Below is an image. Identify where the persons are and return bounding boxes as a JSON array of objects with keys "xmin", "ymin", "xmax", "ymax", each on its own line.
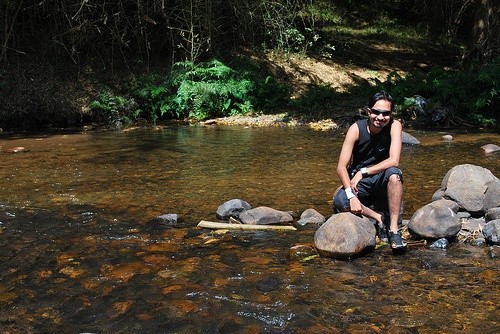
[{"xmin": 332, "ymin": 90, "xmax": 408, "ymax": 250}]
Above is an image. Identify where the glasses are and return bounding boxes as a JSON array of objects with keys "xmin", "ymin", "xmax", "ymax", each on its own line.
[{"xmin": 371, "ymin": 109, "xmax": 391, "ymax": 115}]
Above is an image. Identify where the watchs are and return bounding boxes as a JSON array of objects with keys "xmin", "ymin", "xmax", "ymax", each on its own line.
[{"xmin": 361, "ymin": 167, "xmax": 368, "ymax": 178}]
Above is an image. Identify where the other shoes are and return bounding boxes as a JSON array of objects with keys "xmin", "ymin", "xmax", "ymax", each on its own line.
[
  {"xmin": 378, "ymin": 213, "xmax": 389, "ymax": 242},
  {"xmin": 390, "ymin": 231, "xmax": 407, "ymax": 248}
]
